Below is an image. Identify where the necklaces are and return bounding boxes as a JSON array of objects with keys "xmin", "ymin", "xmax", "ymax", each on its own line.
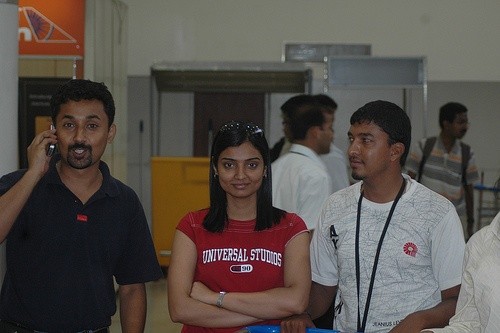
[{"xmin": 355, "ymin": 179, "xmax": 406, "ymax": 333}]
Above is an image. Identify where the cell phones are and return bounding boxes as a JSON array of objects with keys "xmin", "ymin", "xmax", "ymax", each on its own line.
[{"xmin": 46, "ymin": 122, "xmax": 55, "ymax": 156}]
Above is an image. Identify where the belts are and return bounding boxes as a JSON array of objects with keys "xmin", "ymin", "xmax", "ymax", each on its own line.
[{"xmin": 2, "ymin": 320, "xmax": 107, "ymax": 333}]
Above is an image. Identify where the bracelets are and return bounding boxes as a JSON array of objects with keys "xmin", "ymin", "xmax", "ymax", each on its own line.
[
  {"xmin": 217, "ymin": 292, "xmax": 227, "ymax": 308},
  {"xmin": 466, "ymin": 219, "xmax": 475, "ymax": 223}
]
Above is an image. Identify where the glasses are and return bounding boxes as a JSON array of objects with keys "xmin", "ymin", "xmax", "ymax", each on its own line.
[{"xmin": 218, "ymin": 121, "xmax": 263, "ymax": 136}]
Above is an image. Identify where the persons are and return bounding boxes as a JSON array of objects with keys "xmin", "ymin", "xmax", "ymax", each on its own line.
[
  {"xmin": 167, "ymin": 121, "xmax": 311, "ymax": 333},
  {"xmin": 420, "ymin": 211, "xmax": 500, "ymax": 333},
  {"xmin": 272, "ymin": 94, "xmax": 350, "ymax": 241},
  {"xmin": 404, "ymin": 102, "xmax": 479, "ymax": 243},
  {"xmin": 0, "ymin": 79, "xmax": 163, "ymax": 333},
  {"xmin": 281, "ymin": 99, "xmax": 465, "ymax": 333}
]
